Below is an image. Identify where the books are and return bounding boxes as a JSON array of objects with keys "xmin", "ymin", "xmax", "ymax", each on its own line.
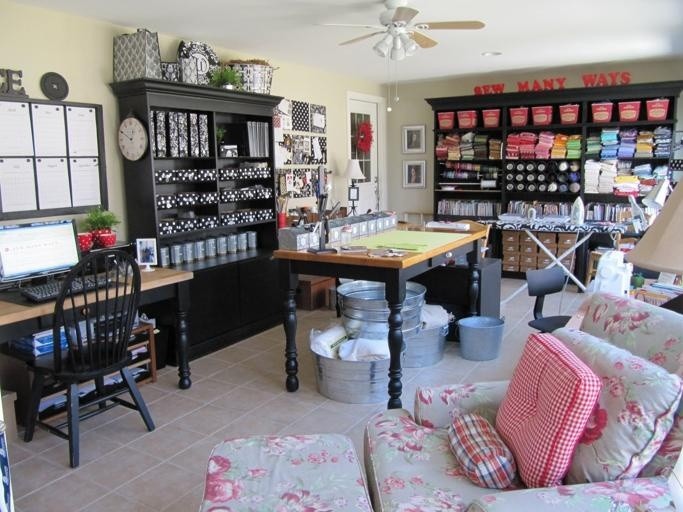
[
  {"xmin": 436, "ymin": 199, "xmax": 658, "ymax": 224},
  {"xmin": 636, "ymin": 282, "xmax": 682, "ymax": 305},
  {"xmin": 237, "ymin": 120, "xmax": 268, "ymax": 158}
]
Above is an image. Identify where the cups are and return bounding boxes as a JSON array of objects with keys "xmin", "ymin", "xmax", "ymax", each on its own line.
[
  {"xmin": 77, "ymin": 232, "xmax": 91, "ymax": 253},
  {"xmin": 277, "ymin": 212, "xmax": 284, "ymax": 228},
  {"xmin": 527, "ymin": 208, "xmax": 536, "ymax": 219}
]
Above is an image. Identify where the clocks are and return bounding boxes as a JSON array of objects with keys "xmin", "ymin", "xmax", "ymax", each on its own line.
[{"xmin": 118, "ymin": 112, "xmax": 148, "ymax": 161}]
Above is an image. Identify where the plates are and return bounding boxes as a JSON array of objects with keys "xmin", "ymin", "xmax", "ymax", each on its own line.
[{"xmin": 497, "ymin": 213, "xmax": 519, "ymax": 222}]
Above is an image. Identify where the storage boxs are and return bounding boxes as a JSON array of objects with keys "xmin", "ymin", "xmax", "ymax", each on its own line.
[{"xmin": 295, "ymin": 273, "xmax": 336, "ymax": 310}]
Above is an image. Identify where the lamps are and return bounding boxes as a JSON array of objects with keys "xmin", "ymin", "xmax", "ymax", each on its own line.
[
  {"xmin": 372, "ymin": 32, "xmax": 419, "ymax": 113},
  {"xmin": 343, "ymin": 160, "xmax": 366, "ymax": 217}
]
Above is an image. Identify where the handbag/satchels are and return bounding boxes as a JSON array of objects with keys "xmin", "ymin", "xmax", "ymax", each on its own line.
[{"xmin": 113, "ymin": 28, "xmax": 162, "ymax": 82}]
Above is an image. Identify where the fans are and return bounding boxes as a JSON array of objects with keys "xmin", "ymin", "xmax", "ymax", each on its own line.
[{"xmin": 324, "ymin": 0, "xmax": 483, "ymax": 48}]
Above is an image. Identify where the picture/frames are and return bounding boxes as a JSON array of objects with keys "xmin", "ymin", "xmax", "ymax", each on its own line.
[
  {"xmin": 402, "ymin": 125, "xmax": 425, "ymax": 154},
  {"xmin": 136, "ymin": 238, "xmax": 157, "ymax": 272},
  {"xmin": 402, "ymin": 160, "xmax": 425, "ymax": 189}
]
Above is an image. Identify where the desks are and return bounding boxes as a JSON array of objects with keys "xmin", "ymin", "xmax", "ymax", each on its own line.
[
  {"xmin": 0, "ymin": 265, "xmax": 194, "ymax": 389},
  {"xmin": 273, "ymin": 223, "xmax": 487, "ymax": 409}
]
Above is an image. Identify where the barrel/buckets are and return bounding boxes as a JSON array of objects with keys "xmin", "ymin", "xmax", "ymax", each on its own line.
[{"xmin": 455, "ymin": 316, "xmax": 507, "ymax": 362}]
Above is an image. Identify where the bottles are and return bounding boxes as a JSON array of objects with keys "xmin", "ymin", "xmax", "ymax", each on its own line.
[
  {"xmin": 572, "ymin": 197, "xmax": 583, "ymax": 225},
  {"xmin": 628, "ymin": 194, "xmax": 647, "ymax": 233},
  {"xmin": 339, "ymin": 227, "xmax": 351, "ymax": 250}
]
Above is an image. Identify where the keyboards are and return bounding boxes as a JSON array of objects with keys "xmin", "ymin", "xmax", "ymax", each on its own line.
[{"xmin": 20, "ymin": 276, "xmax": 111, "ymax": 302}]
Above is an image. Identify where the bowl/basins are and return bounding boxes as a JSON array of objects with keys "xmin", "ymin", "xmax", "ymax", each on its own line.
[
  {"xmin": 292, "ymin": 220, "xmax": 303, "ymax": 227},
  {"xmin": 97, "ymin": 233, "xmax": 116, "ymax": 249}
]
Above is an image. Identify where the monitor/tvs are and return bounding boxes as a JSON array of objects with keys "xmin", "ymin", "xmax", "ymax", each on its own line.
[{"xmin": 0, "ymin": 218, "xmax": 82, "ymax": 292}]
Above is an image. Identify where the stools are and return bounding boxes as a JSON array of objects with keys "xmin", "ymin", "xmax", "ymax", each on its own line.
[{"xmin": 198, "ymin": 433, "xmax": 374, "ymax": 512}]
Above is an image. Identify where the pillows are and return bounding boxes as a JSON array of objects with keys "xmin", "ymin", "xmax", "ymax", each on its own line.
[
  {"xmin": 493, "ymin": 332, "xmax": 602, "ymax": 490},
  {"xmin": 446, "ymin": 412, "xmax": 516, "ymax": 489},
  {"xmin": 551, "ymin": 327, "xmax": 683, "ymax": 485}
]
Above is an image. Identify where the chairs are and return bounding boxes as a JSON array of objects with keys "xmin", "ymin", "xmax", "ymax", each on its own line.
[
  {"xmin": 24, "ymin": 249, "xmax": 155, "ymax": 468},
  {"xmin": 364, "ymin": 292, "xmax": 683, "ymax": 512},
  {"xmin": 524, "ymin": 267, "xmax": 573, "ymax": 331}
]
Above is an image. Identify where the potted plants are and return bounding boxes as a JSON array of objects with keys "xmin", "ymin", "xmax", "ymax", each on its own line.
[
  {"xmin": 80, "ymin": 205, "xmax": 122, "ymax": 245},
  {"xmin": 207, "ymin": 66, "xmax": 244, "ymax": 95}
]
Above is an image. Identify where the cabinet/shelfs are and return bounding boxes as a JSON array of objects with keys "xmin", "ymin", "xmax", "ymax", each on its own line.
[
  {"xmin": 109, "ymin": 77, "xmax": 284, "ymax": 367},
  {"xmin": 424, "ymin": 81, "xmax": 683, "ymax": 303},
  {"xmin": 0, "ymin": 323, "xmax": 157, "ymax": 427},
  {"xmin": 408, "ymin": 257, "xmax": 501, "ymax": 342}
]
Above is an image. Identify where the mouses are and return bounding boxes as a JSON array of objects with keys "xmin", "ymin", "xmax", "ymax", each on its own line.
[{"xmin": 101, "ymin": 275, "xmax": 111, "ymax": 283}]
